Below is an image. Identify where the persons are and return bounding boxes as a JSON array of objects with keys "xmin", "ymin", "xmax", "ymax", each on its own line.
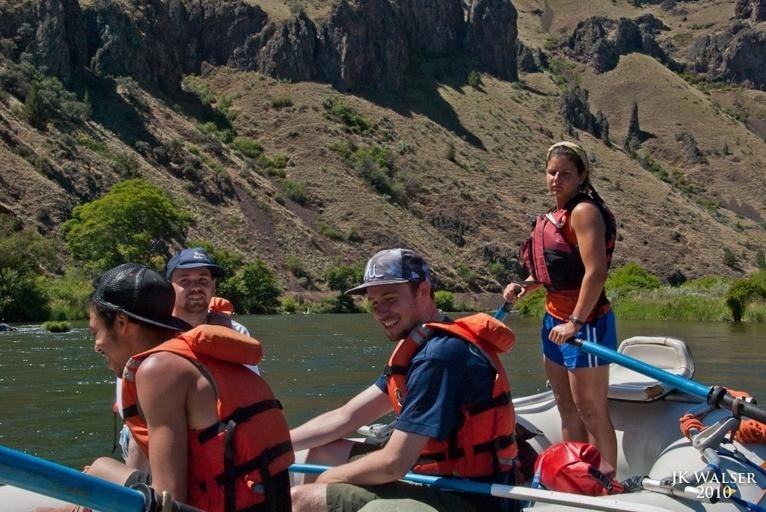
[
  {"xmin": 112, "ymin": 247, "xmax": 261, "ymax": 460},
  {"xmin": 0, "ymin": 259, "xmax": 296, "ymax": 512},
  {"xmin": 290, "ymin": 249, "xmax": 510, "ymax": 512},
  {"xmin": 505, "ymin": 141, "xmax": 617, "ymax": 479}
]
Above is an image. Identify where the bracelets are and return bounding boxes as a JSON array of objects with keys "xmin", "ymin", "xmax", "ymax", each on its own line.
[{"xmin": 568, "ymin": 316, "xmax": 585, "ymax": 326}]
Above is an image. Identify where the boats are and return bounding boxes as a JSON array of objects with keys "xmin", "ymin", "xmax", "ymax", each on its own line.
[{"xmin": 0, "ymin": 336, "xmax": 766, "ymax": 511}]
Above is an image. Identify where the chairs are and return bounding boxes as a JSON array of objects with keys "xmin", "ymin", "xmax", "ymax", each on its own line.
[{"xmin": 608, "ymin": 335, "xmax": 695, "ymax": 402}]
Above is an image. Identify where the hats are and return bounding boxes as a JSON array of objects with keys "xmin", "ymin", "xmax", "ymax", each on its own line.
[
  {"xmin": 167, "ymin": 248, "xmax": 224, "ymax": 280},
  {"xmin": 96, "ymin": 263, "xmax": 193, "ymax": 330},
  {"xmin": 344, "ymin": 248, "xmax": 430, "ymax": 295}
]
